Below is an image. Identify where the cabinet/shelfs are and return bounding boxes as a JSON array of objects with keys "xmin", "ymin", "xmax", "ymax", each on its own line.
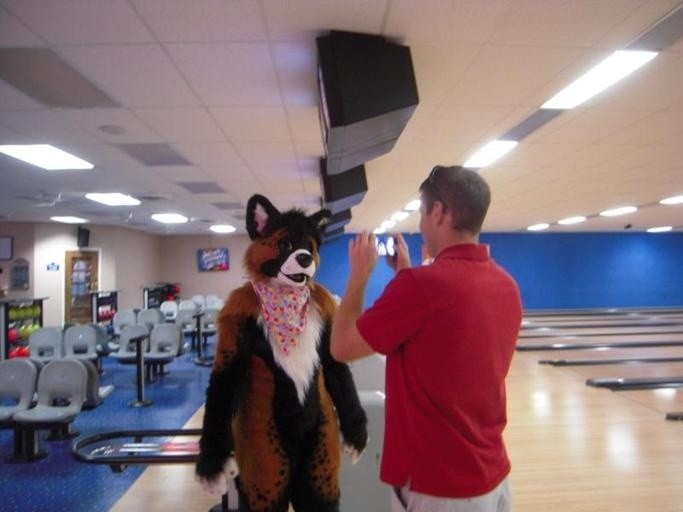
[
  {"xmin": 90, "ymin": 291, "xmax": 118, "ymax": 324},
  {"xmin": 143, "ymin": 284, "xmax": 181, "ymax": 309}
]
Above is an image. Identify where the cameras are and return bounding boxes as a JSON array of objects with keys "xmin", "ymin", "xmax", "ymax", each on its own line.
[{"xmin": 374, "ymin": 234, "xmax": 395, "ymax": 256}]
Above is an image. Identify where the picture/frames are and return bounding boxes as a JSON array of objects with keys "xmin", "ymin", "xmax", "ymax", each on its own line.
[{"xmin": 0, "ymin": 236, "xmax": 13, "ymax": 260}]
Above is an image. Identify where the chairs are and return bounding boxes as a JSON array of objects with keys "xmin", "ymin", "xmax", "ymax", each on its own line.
[{"xmin": 0, "ymin": 295, "xmax": 224, "ymax": 465}]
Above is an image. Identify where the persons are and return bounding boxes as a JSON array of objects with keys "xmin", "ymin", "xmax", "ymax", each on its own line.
[{"xmin": 331, "ymin": 164, "xmax": 525, "ymax": 510}]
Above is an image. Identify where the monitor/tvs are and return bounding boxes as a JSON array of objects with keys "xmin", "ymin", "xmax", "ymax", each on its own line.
[{"xmin": 317, "ymin": 32, "xmax": 421, "ymax": 241}]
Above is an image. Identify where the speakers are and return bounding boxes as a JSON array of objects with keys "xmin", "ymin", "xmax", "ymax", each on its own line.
[{"xmin": 78, "ymin": 228, "xmax": 89, "ymax": 248}]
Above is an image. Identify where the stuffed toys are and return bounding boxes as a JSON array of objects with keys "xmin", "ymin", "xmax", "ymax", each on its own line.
[{"xmin": 193, "ymin": 194, "xmax": 368, "ymax": 512}]
